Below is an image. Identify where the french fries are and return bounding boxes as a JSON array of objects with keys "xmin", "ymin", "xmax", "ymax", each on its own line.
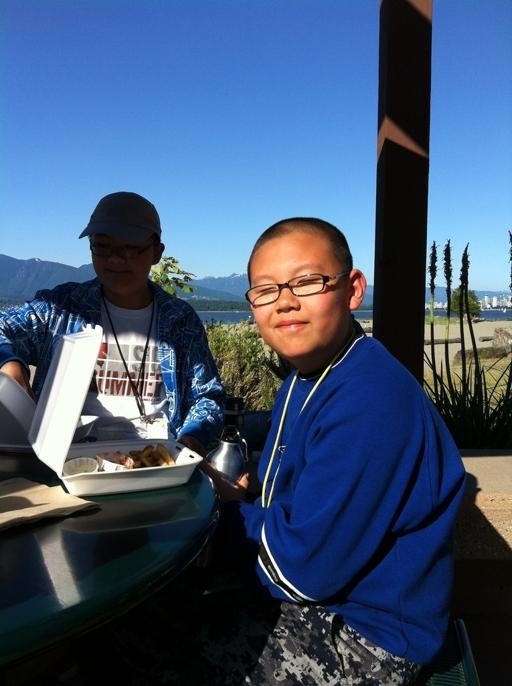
[{"xmin": 129, "ymin": 442, "xmax": 175, "ymax": 468}]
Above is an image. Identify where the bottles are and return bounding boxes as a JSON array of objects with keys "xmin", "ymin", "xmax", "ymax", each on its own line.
[{"xmin": 205, "ymin": 425, "xmax": 248, "ymax": 485}]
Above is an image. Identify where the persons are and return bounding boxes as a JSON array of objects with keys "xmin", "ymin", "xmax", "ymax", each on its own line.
[
  {"xmin": 0, "ymin": 190, "xmax": 230, "ymax": 464},
  {"xmin": 208, "ymin": 216, "xmax": 468, "ymax": 685}
]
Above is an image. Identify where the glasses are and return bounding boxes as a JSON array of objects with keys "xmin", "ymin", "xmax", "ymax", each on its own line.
[
  {"xmin": 88, "ymin": 237, "xmax": 159, "ymax": 258},
  {"xmin": 244, "ymin": 270, "xmax": 353, "ymax": 309}
]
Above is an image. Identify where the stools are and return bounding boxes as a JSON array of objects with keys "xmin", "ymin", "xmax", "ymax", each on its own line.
[{"xmin": 409, "ymin": 619, "xmax": 480, "ymax": 686}]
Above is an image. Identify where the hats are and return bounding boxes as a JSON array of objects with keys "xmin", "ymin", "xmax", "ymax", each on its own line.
[{"xmin": 77, "ymin": 189, "xmax": 164, "ymax": 244}]
